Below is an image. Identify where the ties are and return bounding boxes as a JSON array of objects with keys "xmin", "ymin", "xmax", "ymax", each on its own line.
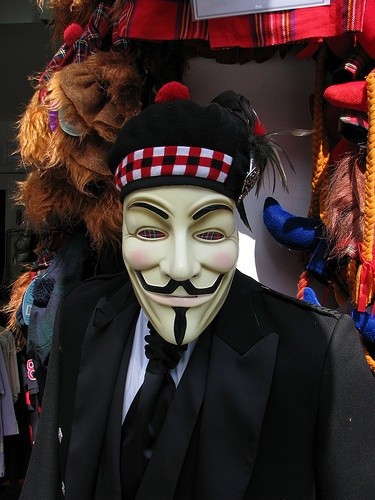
[{"xmin": 119, "ymin": 319, "xmax": 188, "ymax": 499}]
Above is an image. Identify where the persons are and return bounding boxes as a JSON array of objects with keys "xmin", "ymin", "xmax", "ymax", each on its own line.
[{"xmin": 20, "ymin": 82, "xmax": 374, "ymax": 500}]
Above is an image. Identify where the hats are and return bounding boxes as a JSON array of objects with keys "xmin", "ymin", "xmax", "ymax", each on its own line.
[{"xmin": 106, "ymin": 81, "xmax": 298, "ymax": 202}]
